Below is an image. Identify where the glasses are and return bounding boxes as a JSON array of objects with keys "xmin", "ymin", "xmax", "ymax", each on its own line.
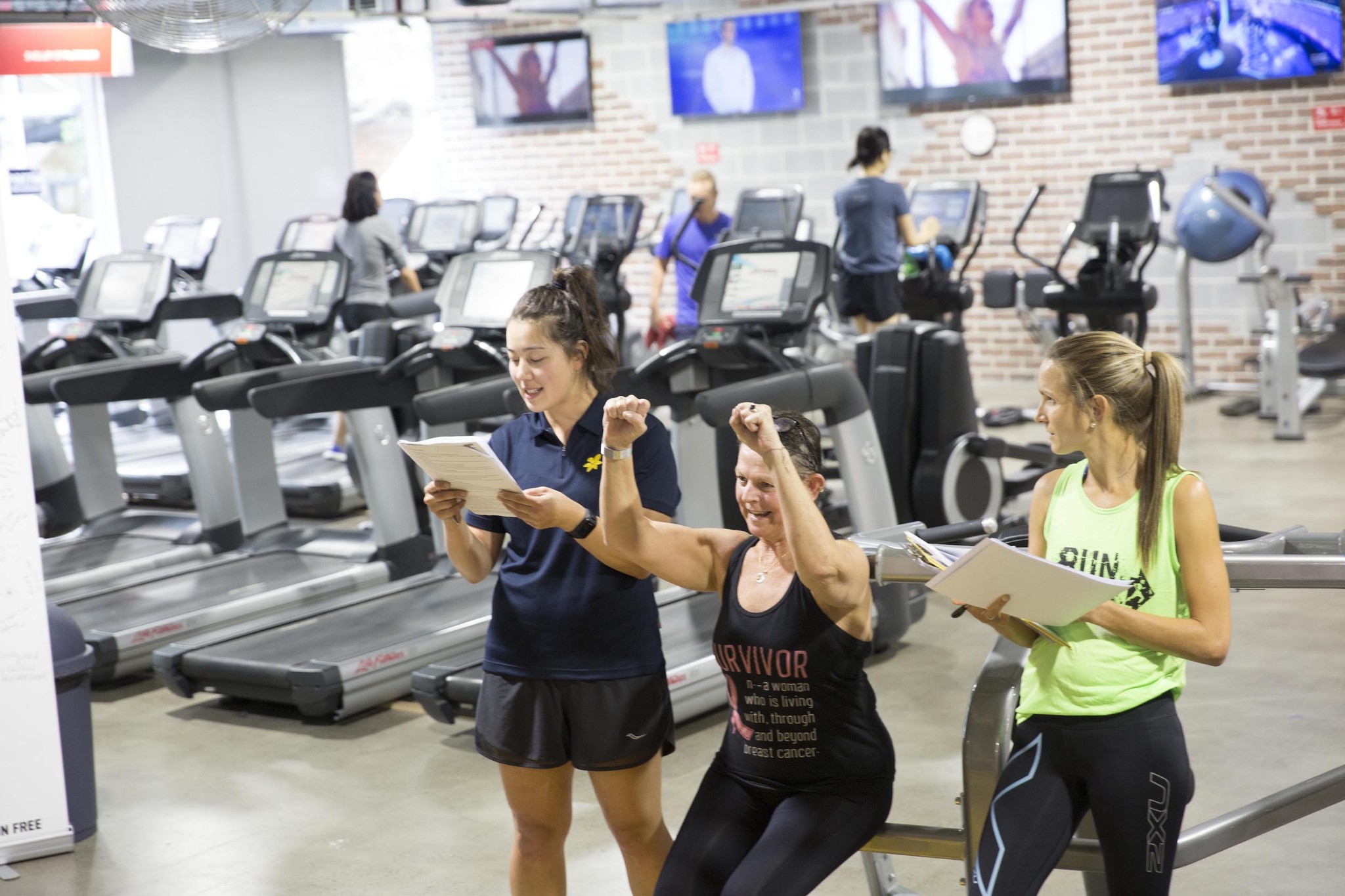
[{"xmin": 774, "ymin": 416, "xmax": 820, "ymax": 470}]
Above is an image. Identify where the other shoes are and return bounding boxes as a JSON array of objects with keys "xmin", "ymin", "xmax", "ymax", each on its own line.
[{"xmin": 324, "ymin": 443, "xmax": 347, "ymax": 462}]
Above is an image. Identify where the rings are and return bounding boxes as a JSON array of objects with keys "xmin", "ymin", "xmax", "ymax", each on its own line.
[{"xmin": 985, "ymin": 615, "xmax": 995, "ymax": 620}]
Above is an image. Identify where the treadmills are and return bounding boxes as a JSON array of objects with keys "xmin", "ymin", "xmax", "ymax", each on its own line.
[
  {"xmin": 14, "ymin": 237, "xmax": 439, "ymax": 688},
  {"xmin": 75, "ymin": 168, "xmax": 1175, "ymax": 551},
  {"xmin": 150, "ymin": 234, "xmax": 630, "ymax": 725},
  {"xmin": 410, "ymin": 231, "xmax": 928, "ymax": 731}
]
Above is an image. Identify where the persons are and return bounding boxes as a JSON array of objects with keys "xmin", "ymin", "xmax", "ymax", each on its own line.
[
  {"xmin": 953, "ymin": 332, "xmax": 1230, "ymax": 896},
  {"xmin": 324, "ymin": 171, "xmax": 421, "ymax": 461},
  {"xmin": 916, "ymin": 0, "xmax": 1025, "ymax": 83},
  {"xmin": 702, "ymin": 21, "xmax": 754, "ymax": 114},
  {"xmin": 835, "ymin": 128, "xmax": 937, "ymax": 338},
  {"xmin": 422, "ymin": 267, "xmax": 683, "ymax": 896},
  {"xmin": 1200, "ymin": 1, "xmax": 1220, "ymax": 51},
  {"xmin": 599, "ymin": 395, "xmax": 895, "ymax": 896},
  {"xmin": 650, "ymin": 170, "xmax": 731, "ymax": 341},
  {"xmin": 489, "ymin": 41, "xmax": 559, "ymax": 114}
]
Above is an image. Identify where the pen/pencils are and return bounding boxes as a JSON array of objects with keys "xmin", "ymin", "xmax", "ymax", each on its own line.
[{"xmin": 951, "ymin": 604, "xmax": 970, "ymax": 618}]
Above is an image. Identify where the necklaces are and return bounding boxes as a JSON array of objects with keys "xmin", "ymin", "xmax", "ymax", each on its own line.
[{"xmin": 755, "ymin": 547, "xmax": 790, "ymax": 583}]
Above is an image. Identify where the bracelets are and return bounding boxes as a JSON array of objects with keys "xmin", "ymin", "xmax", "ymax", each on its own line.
[{"xmin": 601, "ymin": 445, "xmax": 632, "ymax": 460}]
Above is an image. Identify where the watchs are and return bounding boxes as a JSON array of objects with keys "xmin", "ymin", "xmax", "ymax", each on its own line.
[{"xmin": 565, "ymin": 508, "xmax": 596, "ymax": 539}]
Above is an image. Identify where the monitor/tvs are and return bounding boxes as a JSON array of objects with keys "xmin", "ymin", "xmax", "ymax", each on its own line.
[
  {"xmin": 719, "ymin": 250, "xmax": 806, "ymax": 319},
  {"xmin": 470, "ymin": 31, "xmax": 596, "ymax": 126},
  {"xmin": 876, "ymin": 2, "xmax": 1070, "ymax": 107},
  {"xmin": 80, "ymin": 189, "xmax": 548, "ymax": 330},
  {"xmin": 734, "ymin": 188, "xmax": 805, "ymax": 237},
  {"xmin": 571, "ymin": 195, "xmax": 642, "ymax": 253},
  {"xmin": 902, "ymin": 181, "xmax": 980, "ymax": 243},
  {"xmin": 1080, "ymin": 171, "xmax": 1160, "ymax": 238},
  {"xmin": 664, "ymin": 11, "xmax": 806, "ymax": 115}
]
